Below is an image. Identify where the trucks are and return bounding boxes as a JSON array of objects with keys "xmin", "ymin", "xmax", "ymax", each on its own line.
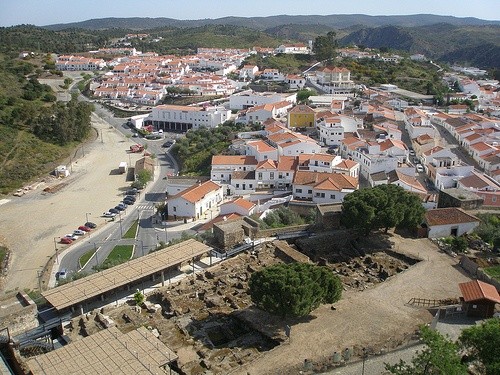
[{"xmin": 119, "ymin": 161, "xmax": 128, "ymax": 173}]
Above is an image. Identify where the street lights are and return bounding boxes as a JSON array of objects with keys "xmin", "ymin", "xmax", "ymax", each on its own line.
[
  {"xmin": 54, "ymin": 237, "xmax": 61, "ymax": 250},
  {"xmin": 86, "ymin": 213, "xmax": 91, "ymax": 222}
]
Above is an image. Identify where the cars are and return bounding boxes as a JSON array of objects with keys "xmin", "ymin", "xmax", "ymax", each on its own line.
[
  {"xmin": 61, "ymin": 238, "xmax": 73, "ymax": 244},
  {"xmin": 64, "ymin": 234, "xmax": 78, "ymax": 240},
  {"xmin": 124, "ymin": 118, "xmax": 167, "ymax": 141},
  {"xmin": 101, "ymin": 94, "xmax": 154, "ymax": 113},
  {"xmin": 72, "ymin": 230, "xmax": 85, "ymax": 236},
  {"xmin": 59, "ymin": 268, "xmax": 68, "ymax": 280},
  {"xmin": 126, "ymin": 185, "xmax": 141, "ymax": 196},
  {"xmin": 129, "ymin": 143, "xmax": 144, "ymax": 153},
  {"xmin": 293, "ymin": 126, "xmax": 341, "ymax": 154},
  {"xmin": 127, "ymin": 195, "xmax": 136, "ymax": 200},
  {"xmin": 85, "ymin": 222, "xmax": 97, "ymax": 228},
  {"xmin": 123, "ymin": 197, "xmax": 135, "ymax": 202},
  {"xmin": 109, "ymin": 209, "xmax": 120, "ymax": 214},
  {"xmin": 123, "ymin": 200, "xmax": 134, "ymax": 205},
  {"xmin": 78, "ymin": 225, "xmax": 91, "ymax": 232},
  {"xmin": 410, "ymin": 149, "xmax": 434, "ymax": 192},
  {"xmin": 118, "ymin": 203, "xmax": 128, "ymax": 208},
  {"xmin": 115, "ymin": 206, "xmax": 125, "ymax": 211},
  {"xmin": 103, "ymin": 212, "xmax": 116, "ymax": 217}
]
